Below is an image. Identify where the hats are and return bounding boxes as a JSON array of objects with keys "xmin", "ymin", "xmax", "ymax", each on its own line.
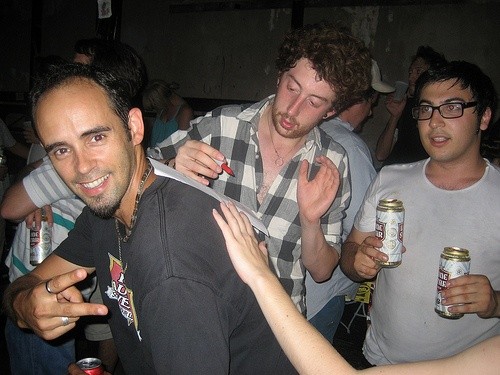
[{"xmin": 368, "ymin": 59, "xmax": 395, "ymax": 93}]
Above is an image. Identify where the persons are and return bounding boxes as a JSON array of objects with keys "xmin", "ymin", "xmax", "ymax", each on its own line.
[{"xmin": 0, "ymin": 25, "xmax": 499, "ymax": 375}]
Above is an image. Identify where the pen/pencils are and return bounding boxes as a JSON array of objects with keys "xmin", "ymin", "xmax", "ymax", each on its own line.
[{"xmin": 210, "ymin": 157, "xmax": 235, "ymax": 177}]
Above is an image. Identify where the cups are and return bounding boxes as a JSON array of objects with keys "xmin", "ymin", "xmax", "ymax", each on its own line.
[{"xmin": 394, "ymin": 80, "xmax": 408, "ymax": 102}]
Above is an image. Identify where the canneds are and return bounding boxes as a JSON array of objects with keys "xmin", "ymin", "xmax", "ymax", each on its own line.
[
  {"xmin": 75, "ymin": 357, "xmax": 104, "ymax": 375},
  {"xmin": 29, "ymin": 214, "xmax": 53, "ymax": 266},
  {"xmin": 373, "ymin": 199, "xmax": 404, "ymax": 268},
  {"xmin": 435, "ymin": 246, "xmax": 471, "ymax": 319}
]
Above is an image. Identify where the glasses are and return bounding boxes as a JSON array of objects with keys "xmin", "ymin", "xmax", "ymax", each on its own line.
[{"xmin": 411, "ymin": 101, "xmax": 478, "ymax": 120}]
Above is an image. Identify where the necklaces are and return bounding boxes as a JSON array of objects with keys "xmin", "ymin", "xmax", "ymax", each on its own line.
[
  {"xmin": 267, "ymin": 107, "xmax": 303, "ymax": 166},
  {"xmin": 116, "ymin": 160, "xmax": 150, "ymax": 243}
]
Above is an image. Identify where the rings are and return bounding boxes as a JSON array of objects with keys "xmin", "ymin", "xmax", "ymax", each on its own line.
[
  {"xmin": 62, "ymin": 316, "xmax": 69, "ymax": 326},
  {"xmin": 45, "ymin": 279, "xmax": 55, "ymax": 293}
]
